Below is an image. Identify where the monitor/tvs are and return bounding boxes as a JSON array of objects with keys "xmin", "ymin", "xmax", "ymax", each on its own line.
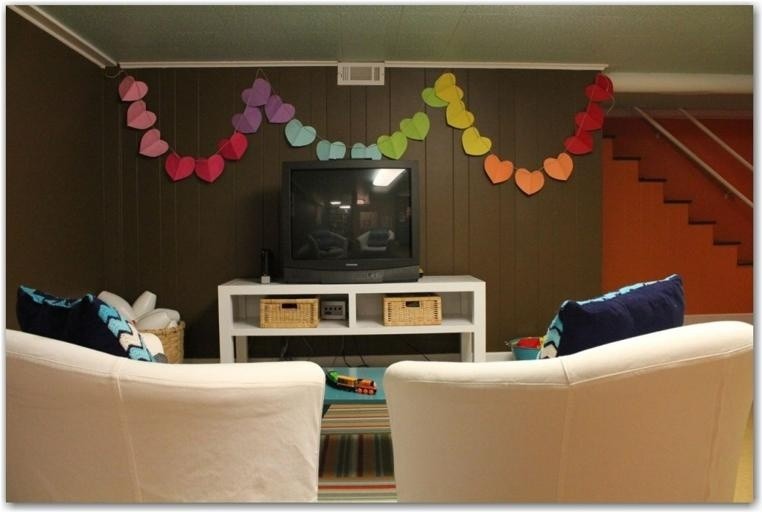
[{"xmin": 280, "ymin": 159, "xmax": 420, "ymax": 284}]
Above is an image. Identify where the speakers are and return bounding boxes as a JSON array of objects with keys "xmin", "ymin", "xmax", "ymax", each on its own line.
[{"xmin": 255, "ymin": 247, "xmax": 271, "ymax": 277}]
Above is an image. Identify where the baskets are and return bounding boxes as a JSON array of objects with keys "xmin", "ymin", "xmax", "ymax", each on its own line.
[
  {"xmin": 258, "ymin": 297, "xmax": 319, "ymax": 328},
  {"xmin": 382, "ymin": 294, "xmax": 441, "ymax": 325},
  {"xmin": 141, "ymin": 318, "xmax": 186, "ymax": 365}
]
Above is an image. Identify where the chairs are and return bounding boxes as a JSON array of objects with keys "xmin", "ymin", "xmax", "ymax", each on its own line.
[
  {"xmin": 5, "ymin": 322, "xmax": 325, "ymax": 501},
  {"xmin": 383, "ymin": 320, "xmax": 756, "ymax": 505}
]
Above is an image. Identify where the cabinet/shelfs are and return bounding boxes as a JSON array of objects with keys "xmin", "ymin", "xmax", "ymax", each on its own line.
[{"xmin": 216, "ymin": 275, "xmax": 490, "ymax": 368}]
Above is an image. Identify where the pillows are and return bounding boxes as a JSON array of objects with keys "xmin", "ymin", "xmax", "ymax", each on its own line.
[
  {"xmin": 535, "ymin": 273, "xmax": 684, "ymax": 363},
  {"xmin": 10, "ymin": 283, "xmax": 160, "ymax": 366}
]
